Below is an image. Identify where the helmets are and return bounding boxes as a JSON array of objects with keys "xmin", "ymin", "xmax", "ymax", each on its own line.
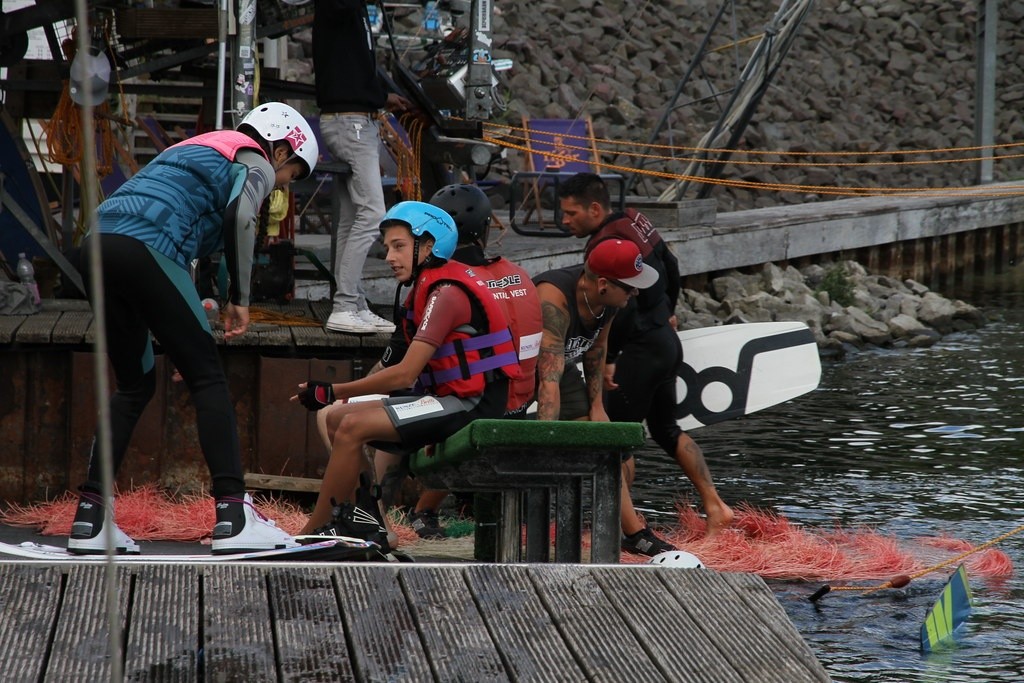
[
  {"xmin": 429, "ymin": 181, "xmax": 491, "ymax": 247},
  {"xmin": 238, "ymin": 102, "xmax": 319, "ymax": 184},
  {"xmin": 379, "ymin": 200, "xmax": 458, "ymax": 264}
]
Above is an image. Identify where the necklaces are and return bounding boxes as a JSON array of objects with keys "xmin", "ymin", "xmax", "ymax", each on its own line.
[{"xmin": 581, "ymin": 274, "xmax": 606, "ymax": 320}]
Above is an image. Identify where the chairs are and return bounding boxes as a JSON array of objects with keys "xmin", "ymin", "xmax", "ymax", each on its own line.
[{"xmin": 0, "ymin": 102, "xmax": 625, "ymax": 298}]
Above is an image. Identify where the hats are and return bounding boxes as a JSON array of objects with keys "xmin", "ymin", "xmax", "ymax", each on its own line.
[
  {"xmin": 70, "ymin": 45, "xmax": 112, "ymax": 107},
  {"xmin": 589, "ymin": 239, "xmax": 659, "ymax": 290}
]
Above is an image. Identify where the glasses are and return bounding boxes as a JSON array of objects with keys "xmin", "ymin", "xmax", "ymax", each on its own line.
[{"xmin": 609, "ymin": 280, "xmax": 633, "ymax": 295}]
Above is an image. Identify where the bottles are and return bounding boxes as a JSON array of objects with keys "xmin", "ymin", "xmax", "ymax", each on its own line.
[
  {"xmin": 16, "ymin": 253, "xmax": 42, "ymax": 306},
  {"xmin": 199, "ymin": 299, "xmax": 221, "ymax": 323}
]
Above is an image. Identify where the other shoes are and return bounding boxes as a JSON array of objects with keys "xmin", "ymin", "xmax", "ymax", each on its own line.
[
  {"xmin": 623, "ymin": 526, "xmax": 678, "ymax": 560},
  {"xmin": 411, "ymin": 512, "xmax": 447, "ymax": 538}
]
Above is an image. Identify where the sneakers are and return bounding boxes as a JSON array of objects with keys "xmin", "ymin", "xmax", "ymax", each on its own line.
[
  {"xmin": 211, "ymin": 495, "xmax": 303, "ymax": 550},
  {"xmin": 326, "ymin": 310, "xmax": 396, "ymax": 334},
  {"xmin": 66, "ymin": 490, "xmax": 142, "ymax": 555}
]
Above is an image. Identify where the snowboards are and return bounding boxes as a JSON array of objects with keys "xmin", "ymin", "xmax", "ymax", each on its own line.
[{"xmin": 0, "ymin": 528, "xmax": 383, "ymax": 563}]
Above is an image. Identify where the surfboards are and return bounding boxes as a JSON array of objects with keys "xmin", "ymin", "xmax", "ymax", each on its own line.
[{"xmin": 347, "ymin": 320, "xmax": 821, "ymax": 439}]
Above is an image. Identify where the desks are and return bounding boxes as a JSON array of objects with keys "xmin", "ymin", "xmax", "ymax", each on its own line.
[{"xmin": 509, "ymin": 170, "xmax": 626, "ymax": 237}]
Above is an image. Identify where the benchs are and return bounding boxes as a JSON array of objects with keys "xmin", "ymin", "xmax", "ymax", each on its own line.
[{"xmin": 407, "ymin": 419, "xmax": 646, "ymax": 565}]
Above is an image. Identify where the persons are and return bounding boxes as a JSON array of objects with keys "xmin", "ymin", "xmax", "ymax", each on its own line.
[
  {"xmin": 559, "ymin": 171, "xmax": 733, "ymax": 540},
  {"xmin": 312, "ymin": 0, "xmax": 413, "ymax": 334},
  {"xmin": 290, "ymin": 200, "xmax": 526, "ymax": 549},
  {"xmin": 68, "ymin": 102, "xmax": 319, "ymax": 556},
  {"xmin": 315, "ymin": 184, "xmax": 680, "ymax": 558}
]
